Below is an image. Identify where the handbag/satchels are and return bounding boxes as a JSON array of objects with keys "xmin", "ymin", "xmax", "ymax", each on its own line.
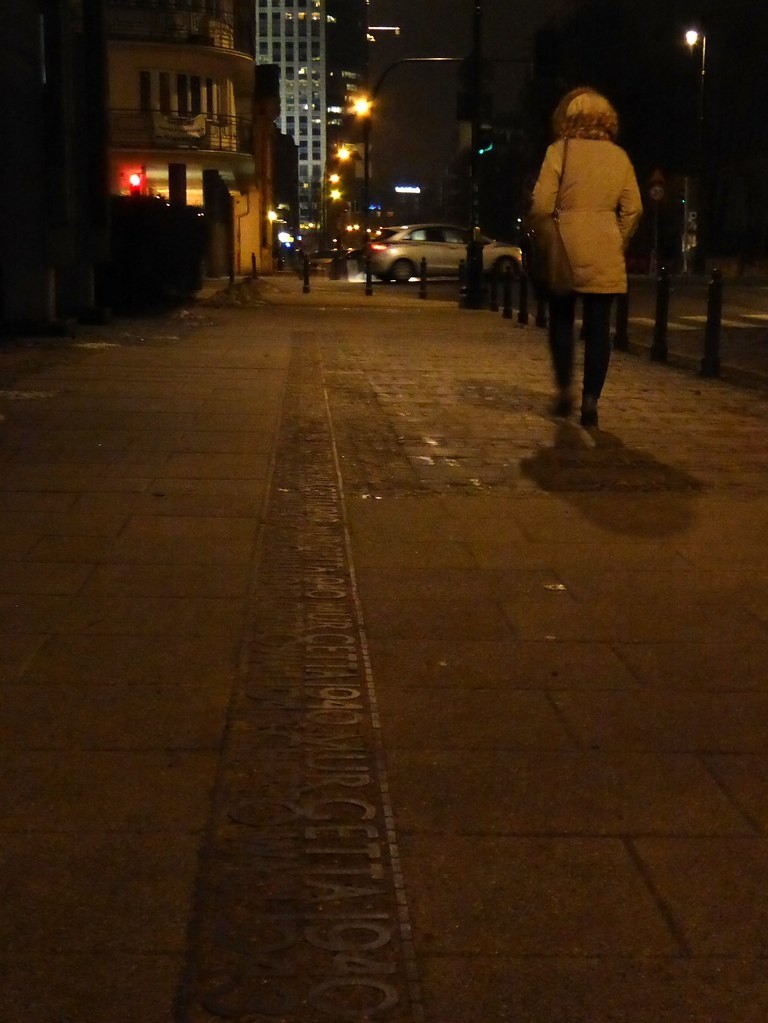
[{"xmin": 517, "ymin": 136, "xmax": 574, "ymax": 297}]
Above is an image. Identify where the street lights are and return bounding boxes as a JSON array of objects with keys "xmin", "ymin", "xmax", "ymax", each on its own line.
[
  {"xmin": 349, "ymin": 93, "xmax": 373, "ymax": 296},
  {"xmin": 336, "ymin": 146, "xmax": 358, "ymax": 258},
  {"xmin": 686, "ymin": 27, "xmax": 708, "ymax": 278}
]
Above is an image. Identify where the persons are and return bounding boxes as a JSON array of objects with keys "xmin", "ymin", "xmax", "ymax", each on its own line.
[{"xmin": 531, "ymin": 87, "xmax": 643, "ymax": 426}]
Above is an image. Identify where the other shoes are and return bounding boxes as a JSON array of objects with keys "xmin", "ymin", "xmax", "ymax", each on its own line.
[
  {"xmin": 552, "ymin": 390, "xmax": 570, "ymax": 416},
  {"xmin": 579, "ymin": 394, "xmax": 599, "ymax": 426}
]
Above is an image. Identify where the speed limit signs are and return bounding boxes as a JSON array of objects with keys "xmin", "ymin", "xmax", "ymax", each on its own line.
[{"xmin": 648, "ymin": 186, "xmax": 665, "ymax": 200}]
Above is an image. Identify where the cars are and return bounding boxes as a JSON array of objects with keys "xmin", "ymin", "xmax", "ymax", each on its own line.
[
  {"xmin": 367, "ymin": 224, "xmax": 522, "ymax": 284},
  {"xmin": 295, "ymin": 247, "xmax": 361, "ymax": 279}
]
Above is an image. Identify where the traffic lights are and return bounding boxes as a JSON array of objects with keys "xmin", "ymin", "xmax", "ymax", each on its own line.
[{"xmin": 478, "ymin": 139, "xmax": 496, "ymax": 162}]
